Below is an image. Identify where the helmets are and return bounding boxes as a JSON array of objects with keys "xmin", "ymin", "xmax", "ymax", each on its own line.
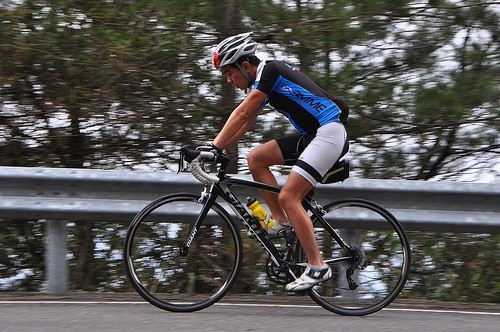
[{"xmin": 214, "ymin": 32, "xmax": 257, "ymax": 69}]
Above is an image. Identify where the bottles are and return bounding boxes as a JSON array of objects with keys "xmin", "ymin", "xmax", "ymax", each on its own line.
[{"xmin": 246, "ymin": 196, "xmax": 274, "ymax": 232}]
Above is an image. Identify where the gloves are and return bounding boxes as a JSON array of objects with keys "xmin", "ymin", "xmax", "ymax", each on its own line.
[{"xmin": 196, "ymin": 143, "xmax": 222, "ymax": 164}]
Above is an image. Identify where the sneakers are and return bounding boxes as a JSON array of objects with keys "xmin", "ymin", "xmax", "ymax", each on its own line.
[
  {"xmin": 247, "ymin": 220, "xmax": 292, "ymax": 238},
  {"xmin": 285, "ymin": 264, "xmax": 332, "ymax": 292}
]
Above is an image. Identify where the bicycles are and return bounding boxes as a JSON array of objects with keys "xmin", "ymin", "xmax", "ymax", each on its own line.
[{"xmin": 123, "ymin": 145, "xmax": 411, "ymax": 316}]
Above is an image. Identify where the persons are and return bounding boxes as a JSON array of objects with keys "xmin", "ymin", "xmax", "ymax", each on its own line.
[{"xmin": 195, "ymin": 32, "xmax": 349, "ymax": 292}]
